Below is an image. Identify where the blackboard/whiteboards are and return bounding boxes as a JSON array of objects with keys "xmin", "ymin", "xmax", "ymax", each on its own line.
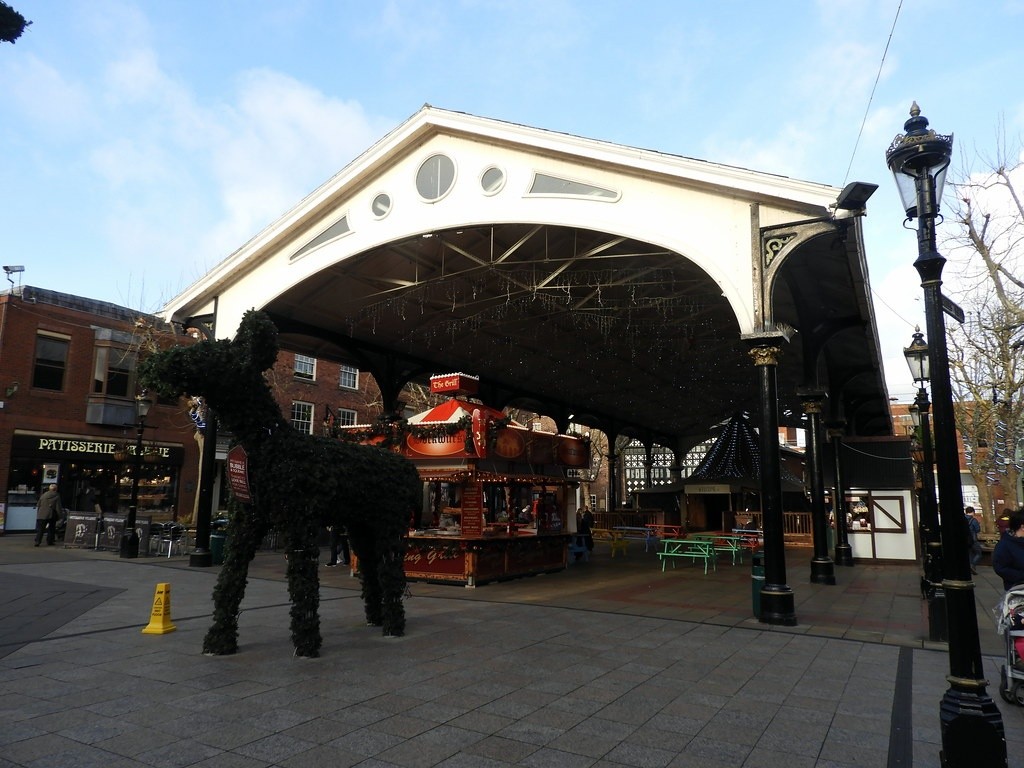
[{"xmin": 536, "ymin": 504, "xmax": 564, "ymax": 536}]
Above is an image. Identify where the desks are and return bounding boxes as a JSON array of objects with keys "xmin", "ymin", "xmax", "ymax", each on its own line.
[{"xmin": 565, "ymin": 523, "xmax": 765, "ymax": 575}]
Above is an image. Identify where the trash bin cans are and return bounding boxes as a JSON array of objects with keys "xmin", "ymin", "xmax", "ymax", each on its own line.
[
  {"xmin": 750, "ymin": 552, "xmax": 766, "ymax": 619},
  {"xmin": 208, "ymin": 520, "xmax": 228, "ymax": 565}
]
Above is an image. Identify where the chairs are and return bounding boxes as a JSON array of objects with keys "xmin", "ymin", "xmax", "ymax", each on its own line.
[{"xmin": 148, "ymin": 521, "xmax": 197, "ymax": 559}]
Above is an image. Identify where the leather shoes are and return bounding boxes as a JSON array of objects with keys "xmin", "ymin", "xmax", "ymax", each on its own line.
[
  {"xmin": 969, "ymin": 565, "xmax": 977, "ymax": 576},
  {"xmin": 34, "ymin": 542, "xmax": 56, "ymax": 546}
]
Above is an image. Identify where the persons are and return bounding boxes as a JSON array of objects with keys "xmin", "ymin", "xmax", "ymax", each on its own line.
[
  {"xmin": 574, "ymin": 508, "xmax": 584, "ymax": 528},
  {"xmin": 325, "ymin": 525, "xmax": 349, "ymax": 567},
  {"xmin": 33, "ymin": 484, "xmax": 63, "ymax": 545},
  {"xmin": 995, "ymin": 508, "xmax": 1015, "ymax": 534},
  {"xmin": 582, "ymin": 505, "xmax": 595, "ymax": 527},
  {"xmin": 962, "ymin": 505, "xmax": 984, "ymax": 574},
  {"xmin": 991, "ymin": 511, "xmax": 1024, "ymax": 593}
]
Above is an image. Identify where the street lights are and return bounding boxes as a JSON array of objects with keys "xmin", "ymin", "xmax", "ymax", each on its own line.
[
  {"xmin": 906, "ymin": 398, "xmax": 935, "ymax": 599},
  {"xmin": 115, "ymin": 388, "xmax": 154, "ymax": 557},
  {"xmin": 903, "ymin": 327, "xmax": 950, "ymax": 638},
  {"xmin": 888, "ymin": 101, "xmax": 1010, "ymax": 768}
]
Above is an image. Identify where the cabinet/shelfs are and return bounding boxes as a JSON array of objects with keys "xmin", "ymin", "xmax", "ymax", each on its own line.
[{"xmin": 118, "ymin": 477, "xmax": 176, "ymax": 516}]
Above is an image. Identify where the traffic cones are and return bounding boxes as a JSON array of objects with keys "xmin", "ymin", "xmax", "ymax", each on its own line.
[{"xmin": 142, "ymin": 582, "xmax": 178, "ymax": 634}]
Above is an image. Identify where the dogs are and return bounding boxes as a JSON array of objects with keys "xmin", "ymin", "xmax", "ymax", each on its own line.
[{"xmin": 137, "ymin": 310, "xmax": 420, "ymax": 658}]
